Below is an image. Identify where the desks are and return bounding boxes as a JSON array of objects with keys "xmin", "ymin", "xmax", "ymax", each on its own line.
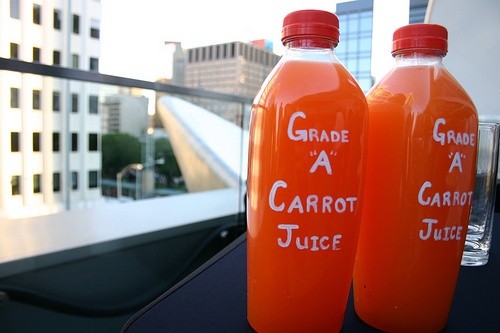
[{"xmin": 119, "ymin": 174, "xmax": 500, "ymax": 333}]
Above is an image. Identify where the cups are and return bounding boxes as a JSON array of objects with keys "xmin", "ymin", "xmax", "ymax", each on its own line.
[{"xmin": 460, "ymin": 123, "xmax": 499, "ymax": 267}]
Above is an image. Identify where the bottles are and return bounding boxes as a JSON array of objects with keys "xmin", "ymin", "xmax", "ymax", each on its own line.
[
  {"xmin": 246, "ymin": 8, "xmax": 370, "ymax": 333},
  {"xmin": 351, "ymin": 23, "xmax": 479, "ymax": 333}
]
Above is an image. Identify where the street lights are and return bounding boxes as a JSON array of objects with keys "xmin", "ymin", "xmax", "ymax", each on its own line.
[{"xmin": 116, "ymin": 163, "xmax": 143, "ymax": 203}]
